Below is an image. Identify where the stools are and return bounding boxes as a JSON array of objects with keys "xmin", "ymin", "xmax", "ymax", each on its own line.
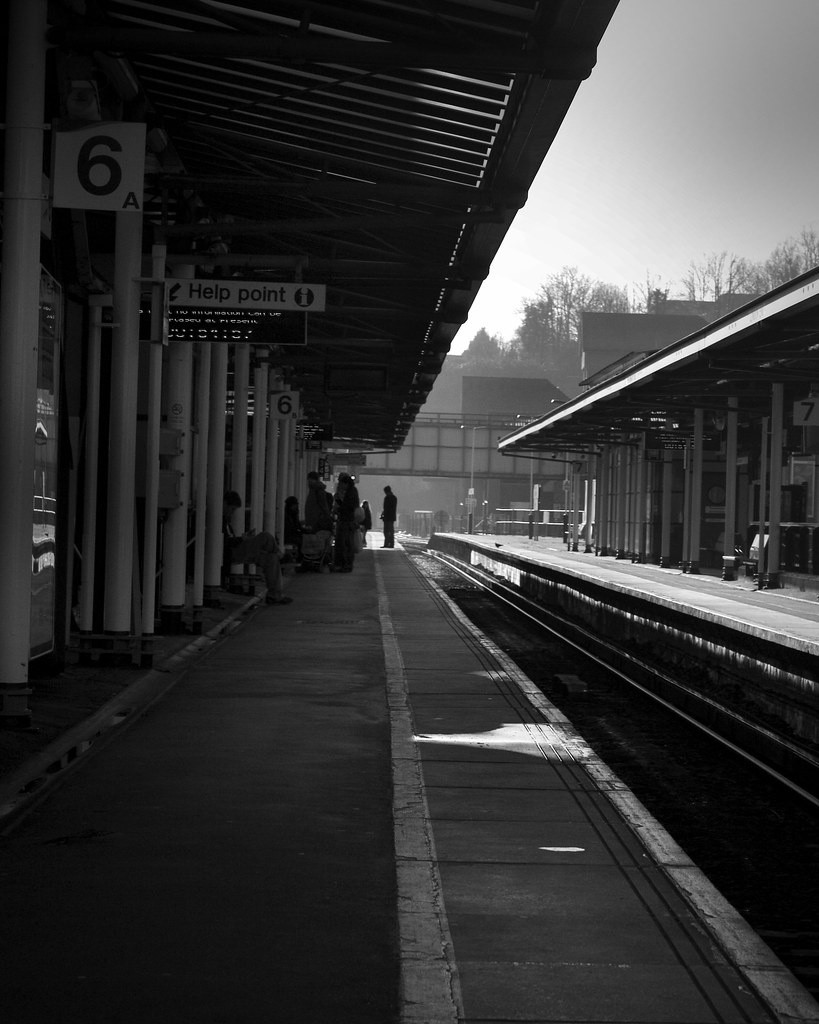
[{"xmin": 228, "ymin": 573, "xmax": 262, "ymax": 596}]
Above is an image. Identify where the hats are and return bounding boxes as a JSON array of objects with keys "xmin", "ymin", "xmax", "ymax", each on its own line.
[{"xmin": 286, "ymin": 496, "xmax": 299, "ymax": 505}]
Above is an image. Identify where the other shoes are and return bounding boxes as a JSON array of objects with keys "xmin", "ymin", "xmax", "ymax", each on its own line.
[
  {"xmin": 266, "ymin": 595, "xmax": 293, "ymax": 605},
  {"xmin": 330, "ymin": 564, "xmax": 351, "ymax": 573}
]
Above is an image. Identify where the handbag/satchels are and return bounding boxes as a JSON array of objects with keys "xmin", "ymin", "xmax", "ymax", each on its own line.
[{"xmin": 354, "ymin": 508, "xmax": 365, "ymax": 523}]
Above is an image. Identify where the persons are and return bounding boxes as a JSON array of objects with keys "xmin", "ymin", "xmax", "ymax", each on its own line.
[
  {"xmin": 362, "ymin": 501, "xmax": 372, "ymax": 546},
  {"xmin": 380, "ymin": 485, "xmax": 397, "ymax": 548},
  {"xmin": 330, "ymin": 471, "xmax": 358, "ymax": 573},
  {"xmin": 284, "ymin": 496, "xmax": 300, "ymax": 550},
  {"xmin": 304, "ymin": 471, "xmax": 333, "ymax": 531},
  {"xmin": 223, "ymin": 491, "xmax": 295, "ymax": 604}
]
